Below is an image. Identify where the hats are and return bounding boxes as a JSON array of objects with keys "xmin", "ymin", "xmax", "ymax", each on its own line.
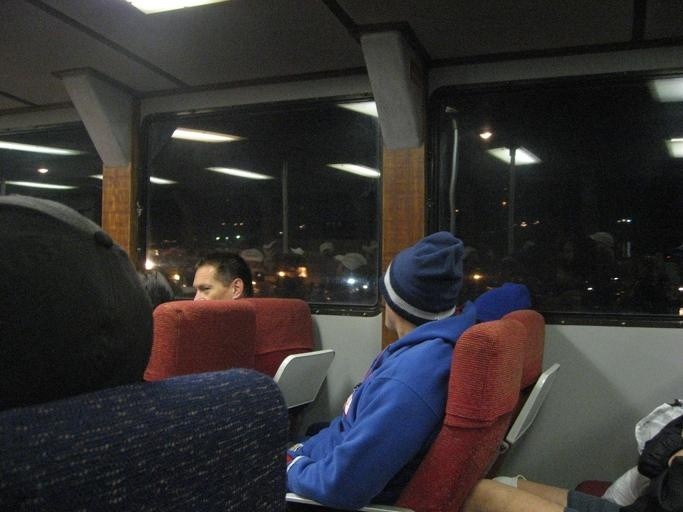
[{"xmin": 380, "ymin": 231, "xmax": 466, "ymax": 324}]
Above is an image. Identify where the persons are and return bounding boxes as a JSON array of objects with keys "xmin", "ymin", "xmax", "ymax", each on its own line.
[
  {"xmin": 0, "ymin": 188, "xmax": 153, "ymax": 410},
  {"xmin": 285, "ymin": 231, "xmax": 532, "ymax": 511},
  {"xmin": 192, "ymin": 251, "xmax": 254, "ymax": 300},
  {"xmin": 138, "ymin": 270, "xmax": 175, "ymax": 307},
  {"xmin": 460, "ymin": 415, "xmax": 683, "ymax": 511}
]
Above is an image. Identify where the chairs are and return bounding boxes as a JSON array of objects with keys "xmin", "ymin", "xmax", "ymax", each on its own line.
[{"xmin": 0, "ymin": 295, "xmax": 551, "ymax": 512}]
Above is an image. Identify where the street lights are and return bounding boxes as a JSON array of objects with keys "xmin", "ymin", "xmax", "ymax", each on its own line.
[{"xmin": 479, "ymin": 130, "xmax": 515, "ymax": 257}]
